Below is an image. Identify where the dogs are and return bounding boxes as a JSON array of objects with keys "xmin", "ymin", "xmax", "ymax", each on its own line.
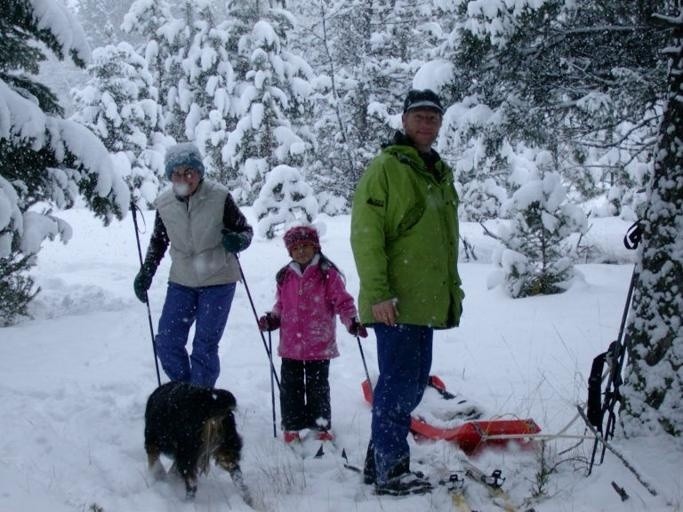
[{"xmin": 142, "ymin": 379, "xmax": 255, "ymax": 512}]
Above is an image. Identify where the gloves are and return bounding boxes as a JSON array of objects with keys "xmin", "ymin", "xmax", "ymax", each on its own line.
[
  {"xmin": 133, "ymin": 263, "xmax": 157, "ymax": 303},
  {"xmin": 260, "ymin": 316, "xmax": 277, "ymax": 331},
  {"xmin": 222, "ymin": 231, "xmax": 245, "ymax": 253},
  {"xmin": 349, "ymin": 322, "xmax": 368, "ymax": 337}
]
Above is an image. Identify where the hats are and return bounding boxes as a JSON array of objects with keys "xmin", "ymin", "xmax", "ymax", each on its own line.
[
  {"xmin": 165, "ymin": 143, "xmax": 205, "ymax": 181},
  {"xmin": 404, "ymin": 89, "xmax": 444, "ymax": 115},
  {"xmin": 283, "ymin": 225, "xmax": 320, "ymax": 257}
]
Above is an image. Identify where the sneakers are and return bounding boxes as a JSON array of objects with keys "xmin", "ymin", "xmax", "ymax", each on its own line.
[
  {"xmin": 283, "ymin": 431, "xmax": 304, "ymax": 448},
  {"xmin": 316, "ymin": 431, "xmax": 336, "ymax": 441},
  {"xmin": 364, "ymin": 471, "xmax": 434, "ymax": 496}
]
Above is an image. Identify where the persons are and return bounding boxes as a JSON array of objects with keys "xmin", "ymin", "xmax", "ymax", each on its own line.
[
  {"xmin": 133, "ymin": 141, "xmax": 254, "ymax": 389},
  {"xmin": 259, "ymin": 226, "xmax": 368, "ymax": 445},
  {"xmin": 350, "ymin": 90, "xmax": 464, "ymax": 496}
]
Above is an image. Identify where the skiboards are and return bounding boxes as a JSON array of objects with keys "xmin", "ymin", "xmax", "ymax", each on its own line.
[
  {"xmin": 288, "ymin": 430, "xmax": 361, "ymax": 475},
  {"xmin": 429, "ymin": 451, "xmax": 523, "ymax": 508}
]
